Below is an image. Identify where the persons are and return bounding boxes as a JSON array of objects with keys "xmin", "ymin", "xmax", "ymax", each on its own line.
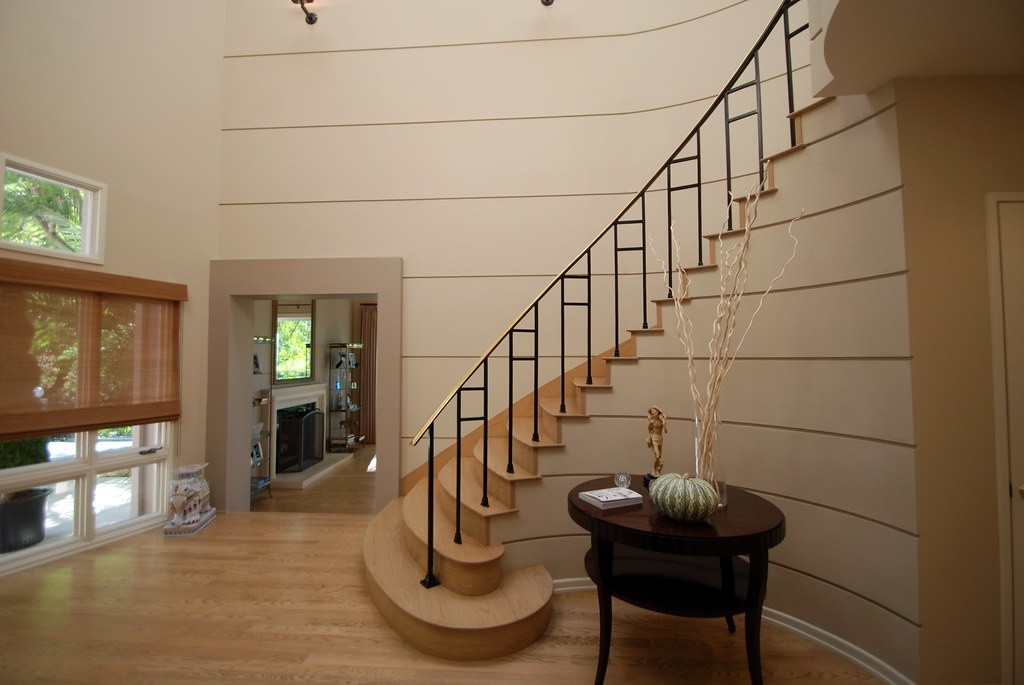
[{"xmin": 647, "ymin": 406, "xmax": 668, "ymax": 477}]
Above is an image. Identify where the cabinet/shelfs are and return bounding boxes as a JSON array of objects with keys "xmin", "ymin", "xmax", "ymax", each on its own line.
[
  {"xmin": 251, "ymin": 336, "xmax": 275, "ymax": 505},
  {"xmin": 329, "ymin": 343, "xmax": 365, "ymax": 454}
]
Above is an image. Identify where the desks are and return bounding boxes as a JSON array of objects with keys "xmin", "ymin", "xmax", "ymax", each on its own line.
[{"xmin": 568, "ymin": 474, "xmax": 786, "ymax": 685}]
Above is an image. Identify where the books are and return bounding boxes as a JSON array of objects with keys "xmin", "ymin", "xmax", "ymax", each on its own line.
[{"xmin": 579, "ymin": 486, "xmax": 643, "ymax": 510}]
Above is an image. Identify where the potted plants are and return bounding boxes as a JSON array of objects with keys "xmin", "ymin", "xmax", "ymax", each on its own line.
[{"xmin": 0, "ymin": 434, "xmax": 53, "ymax": 554}]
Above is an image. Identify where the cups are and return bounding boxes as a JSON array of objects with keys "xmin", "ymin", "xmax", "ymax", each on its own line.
[{"xmin": 614, "ymin": 472, "xmax": 631, "ymax": 488}]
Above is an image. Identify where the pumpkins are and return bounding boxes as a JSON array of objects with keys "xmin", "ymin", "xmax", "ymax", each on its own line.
[{"xmin": 649, "ymin": 472, "xmax": 718, "ymax": 522}]
